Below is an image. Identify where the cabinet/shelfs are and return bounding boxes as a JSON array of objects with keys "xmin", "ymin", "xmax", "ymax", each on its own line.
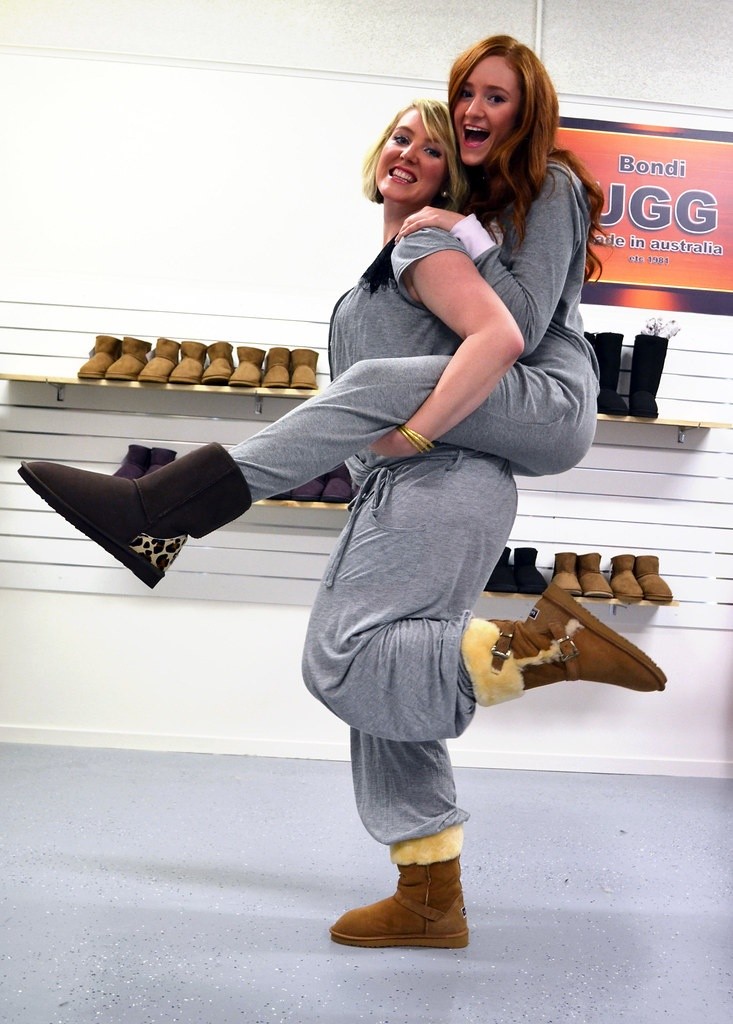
[{"xmin": 0, "ymin": 296, "xmax": 733, "ymax": 780}]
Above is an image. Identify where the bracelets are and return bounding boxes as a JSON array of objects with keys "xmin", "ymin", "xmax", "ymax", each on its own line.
[{"xmin": 398, "ymin": 425, "xmax": 436, "ymax": 454}]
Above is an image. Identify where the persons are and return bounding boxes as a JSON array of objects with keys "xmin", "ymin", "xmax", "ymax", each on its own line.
[
  {"xmin": 17, "ymin": 33, "xmax": 605, "ymax": 587},
  {"xmin": 300, "ymin": 98, "xmax": 668, "ymax": 949}
]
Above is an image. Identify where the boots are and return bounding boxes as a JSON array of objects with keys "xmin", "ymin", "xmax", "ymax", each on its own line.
[
  {"xmin": 168, "ymin": 341, "xmax": 207, "ymax": 385},
  {"xmin": 634, "ymin": 556, "xmax": 673, "ymax": 601},
  {"xmin": 460, "ymin": 585, "xmax": 667, "ymax": 707},
  {"xmin": 630, "ymin": 335, "xmax": 667, "ymax": 418},
  {"xmin": 551, "ymin": 553, "xmax": 582, "ymax": 596},
  {"xmin": 329, "ymin": 822, "xmax": 470, "ymax": 948},
  {"xmin": 261, "ymin": 347, "xmax": 291, "ymax": 388},
  {"xmin": 265, "ymin": 464, "xmax": 353, "ymax": 503},
  {"xmin": 583, "ymin": 331, "xmax": 630, "ymax": 415},
  {"xmin": 577, "ymin": 553, "xmax": 613, "ymax": 597},
  {"xmin": 113, "ymin": 444, "xmax": 177, "ymax": 480},
  {"xmin": 483, "ymin": 548, "xmax": 517, "ymax": 592},
  {"xmin": 513, "ymin": 548, "xmax": 548, "ymax": 594},
  {"xmin": 106, "ymin": 337, "xmax": 151, "ymax": 380},
  {"xmin": 229, "ymin": 347, "xmax": 266, "ymax": 387},
  {"xmin": 290, "ymin": 349, "xmax": 319, "ymax": 389},
  {"xmin": 610, "ymin": 555, "xmax": 644, "ymax": 599},
  {"xmin": 78, "ymin": 335, "xmax": 122, "ymax": 379},
  {"xmin": 138, "ymin": 338, "xmax": 180, "ymax": 383},
  {"xmin": 201, "ymin": 342, "xmax": 234, "ymax": 384},
  {"xmin": 18, "ymin": 443, "xmax": 252, "ymax": 590}
]
